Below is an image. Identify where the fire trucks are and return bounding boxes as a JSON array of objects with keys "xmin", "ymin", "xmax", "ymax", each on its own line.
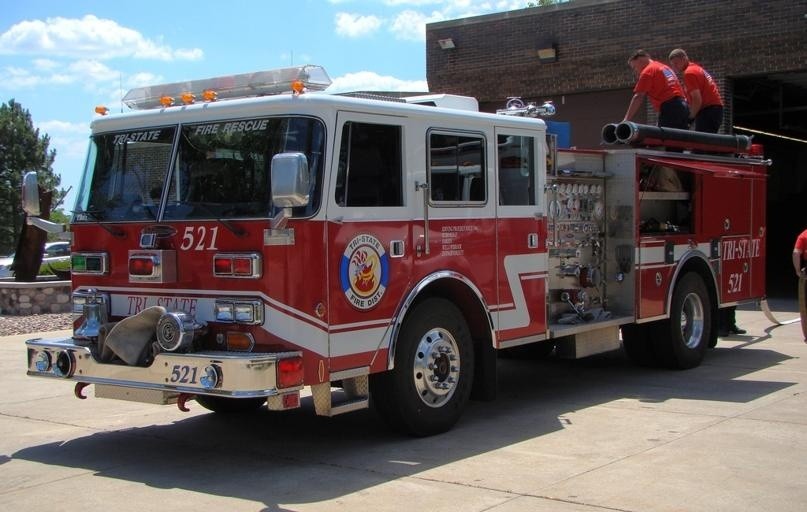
[{"xmin": 22, "ymin": 64, "xmax": 772, "ymax": 438}]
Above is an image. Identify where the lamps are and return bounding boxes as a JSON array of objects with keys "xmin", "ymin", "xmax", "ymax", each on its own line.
[
  {"xmin": 538, "ymin": 44, "xmax": 556, "ymax": 62},
  {"xmin": 439, "ymin": 38, "xmax": 455, "ymax": 51}
]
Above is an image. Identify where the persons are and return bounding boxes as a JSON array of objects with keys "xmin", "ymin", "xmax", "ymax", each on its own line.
[
  {"xmin": 791, "ymin": 229, "xmax": 806, "ymax": 342},
  {"xmin": 716, "ymin": 305, "xmax": 746, "ymax": 337},
  {"xmin": 622, "ymin": 49, "xmax": 690, "ymax": 155},
  {"xmin": 668, "ymin": 48, "xmax": 726, "ymax": 155}
]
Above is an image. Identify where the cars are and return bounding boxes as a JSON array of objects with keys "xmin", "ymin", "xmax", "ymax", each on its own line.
[{"xmin": 0, "ymin": 242, "xmax": 70, "ymax": 277}]
